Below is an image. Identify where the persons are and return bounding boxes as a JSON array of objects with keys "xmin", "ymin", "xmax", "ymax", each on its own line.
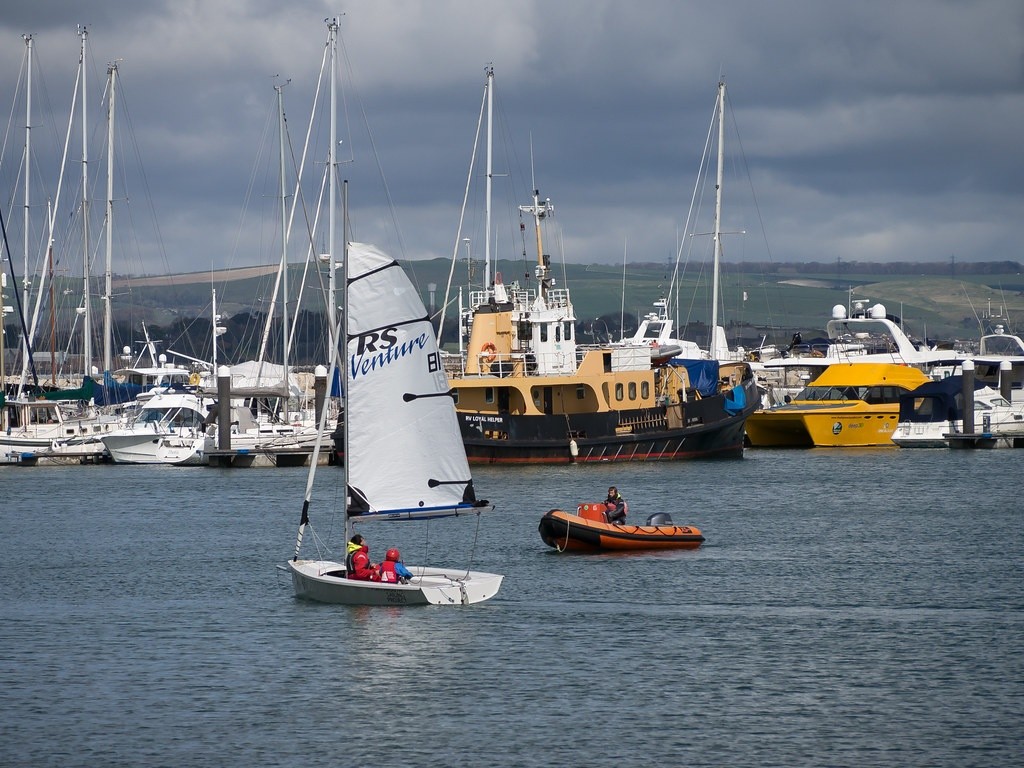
[
  {"xmin": 347, "ymin": 534, "xmax": 413, "ymax": 584},
  {"xmin": 602, "ymin": 486, "xmax": 628, "ymax": 525}
]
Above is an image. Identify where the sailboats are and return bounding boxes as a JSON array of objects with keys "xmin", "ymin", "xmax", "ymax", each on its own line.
[
  {"xmin": 276, "ymin": 182, "xmax": 505, "ymax": 605},
  {"xmin": 1, "ymin": 13, "xmax": 784, "ymax": 467}
]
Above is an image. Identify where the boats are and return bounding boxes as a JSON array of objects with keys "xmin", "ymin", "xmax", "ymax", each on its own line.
[
  {"xmin": 538, "ymin": 504, "xmax": 706, "ymax": 553},
  {"xmin": 890, "ymin": 375, "xmax": 1024, "ymax": 449},
  {"xmin": 744, "ymin": 282, "xmax": 1023, "ymax": 446}
]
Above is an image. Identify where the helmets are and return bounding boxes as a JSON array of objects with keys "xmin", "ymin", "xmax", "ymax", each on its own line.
[{"xmin": 386, "ymin": 548, "xmax": 400, "ymax": 561}]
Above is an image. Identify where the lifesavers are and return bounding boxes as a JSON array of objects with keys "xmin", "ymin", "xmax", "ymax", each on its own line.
[
  {"xmin": 190, "ymin": 373, "xmax": 200, "ymax": 385},
  {"xmin": 481, "ymin": 342, "xmax": 497, "ymax": 362}
]
